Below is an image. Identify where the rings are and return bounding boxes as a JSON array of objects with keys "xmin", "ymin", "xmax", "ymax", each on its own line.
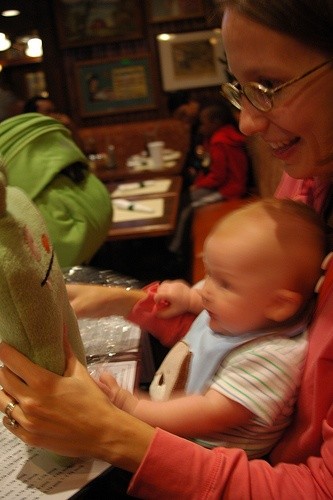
[{"xmin": 6, "ymin": 400, "xmax": 18, "ymax": 428}]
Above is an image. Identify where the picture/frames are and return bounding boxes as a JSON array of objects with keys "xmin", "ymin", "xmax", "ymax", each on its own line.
[
  {"xmin": 158, "ymin": 31, "xmax": 229, "ymax": 93},
  {"xmin": 146, "ymin": 0, "xmax": 206, "ymax": 24},
  {"xmin": 56, "ymin": 0, "xmax": 143, "ymax": 49},
  {"xmin": 73, "ymin": 53, "xmax": 159, "ymax": 118}
]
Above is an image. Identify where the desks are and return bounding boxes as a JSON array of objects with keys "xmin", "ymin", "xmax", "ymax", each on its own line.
[
  {"xmin": 103, "ymin": 177, "xmax": 184, "ymax": 242},
  {"xmin": 74, "ymin": 118, "xmax": 191, "ymax": 181}
]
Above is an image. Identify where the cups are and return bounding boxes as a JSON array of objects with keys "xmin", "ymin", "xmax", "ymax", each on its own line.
[{"xmin": 148, "ymin": 142, "xmax": 164, "ymax": 159}]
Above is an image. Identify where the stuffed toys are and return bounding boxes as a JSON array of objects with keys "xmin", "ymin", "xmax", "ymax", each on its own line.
[{"xmin": 0, "ymin": 159, "xmax": 90, "ymax": 461}]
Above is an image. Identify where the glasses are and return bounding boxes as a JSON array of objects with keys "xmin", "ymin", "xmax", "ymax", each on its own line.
[{"xmin": 219, "ymin": 59, "xmax": 331, "ymax": 112}]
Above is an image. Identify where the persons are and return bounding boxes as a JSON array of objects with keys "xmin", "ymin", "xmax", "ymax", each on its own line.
[
  {"xmin": 169, "ymin": 104, "xmax": 248, "ymax": 253},
  {"xmin": 92, "ymin": 198, "xmax": 326, "ymax": 459},
  {"xmin": 0, "ymin": 0, "xmax": 333, "ymax": 500},
  {"xmin": 85, "ymin": 71, "xmax": 104, "ymax": 103},
  {"xmin": 168, "ymin": 90, "xmax": 238, "ymax": 239},
  {"xmin": 24, "ymin": 92, "xmax": 81, "ymax": 153}
]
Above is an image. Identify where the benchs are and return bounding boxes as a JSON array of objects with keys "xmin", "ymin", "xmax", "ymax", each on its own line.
[{"xmin": 193, "ymin": 110, "xmax": 284, "ymax": 286}]
